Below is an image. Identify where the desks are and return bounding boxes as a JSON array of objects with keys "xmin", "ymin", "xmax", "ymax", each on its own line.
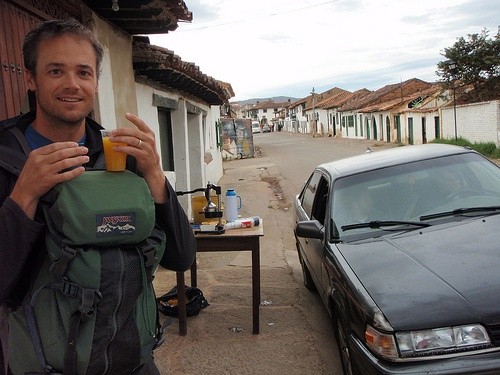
[{"xmin": 176, "ymin": 218, "xmax": 265, "ymax": 339}]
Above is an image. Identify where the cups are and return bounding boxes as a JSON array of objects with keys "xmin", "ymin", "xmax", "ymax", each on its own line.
[{"xmin": 100, "ymin": 129, "xmax": 127, "ymax": 171}]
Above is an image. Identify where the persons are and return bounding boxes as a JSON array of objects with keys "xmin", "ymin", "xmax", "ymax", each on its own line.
[
  {"xmin": 0, "ymin": 19, "xmax": 198, "ymax": 375},
  {"xmin": 335, "ymin": 184, "xmax": 387, "ymax": 224},
  {"xmin": 425, "ymin": 167, "xmax": 479, "ymax": 209}
]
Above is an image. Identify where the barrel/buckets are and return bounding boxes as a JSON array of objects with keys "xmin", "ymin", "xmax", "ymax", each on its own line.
[{"xmin": 192, "ymin": 196, "xmax": 218, "ymax": 225}]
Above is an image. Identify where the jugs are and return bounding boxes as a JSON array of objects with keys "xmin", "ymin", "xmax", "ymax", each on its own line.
[{"xmin": 224, "ymin": 188, "xmax": 243, "ymax": 223}]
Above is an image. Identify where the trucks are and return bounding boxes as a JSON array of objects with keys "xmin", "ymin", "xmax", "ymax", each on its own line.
[{"xmin": 251, "ymin": 120, "xmax": 261, "ymax": 134}]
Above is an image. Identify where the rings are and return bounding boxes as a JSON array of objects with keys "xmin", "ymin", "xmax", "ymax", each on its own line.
[{"xmin": 135, "ymin": 140, "xmax": 143, "ymax": 147}]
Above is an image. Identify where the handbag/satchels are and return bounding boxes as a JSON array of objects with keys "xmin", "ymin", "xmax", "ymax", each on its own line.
[{"xmin": 159, "ymin": 284, "xmax": 205, "ymax": 317}]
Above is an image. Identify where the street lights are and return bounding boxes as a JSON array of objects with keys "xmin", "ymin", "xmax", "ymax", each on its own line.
[{"xmin": 447, "ymin": 60, "xmax": 459, "ymax": 140}]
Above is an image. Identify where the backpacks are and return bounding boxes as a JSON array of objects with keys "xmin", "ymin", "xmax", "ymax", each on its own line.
[{"xmin": 2, "ymin": 129, "xmax": 157, "ymax": 374}]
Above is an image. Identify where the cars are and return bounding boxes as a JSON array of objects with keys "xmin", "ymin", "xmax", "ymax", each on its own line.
[
  {"xmin": 293, "ymin": 142, "xmax": 500, "ymax": 375},
  {"xmin": 262, "ymin": 125, "xmax": 270, "ymax": 133}
]
